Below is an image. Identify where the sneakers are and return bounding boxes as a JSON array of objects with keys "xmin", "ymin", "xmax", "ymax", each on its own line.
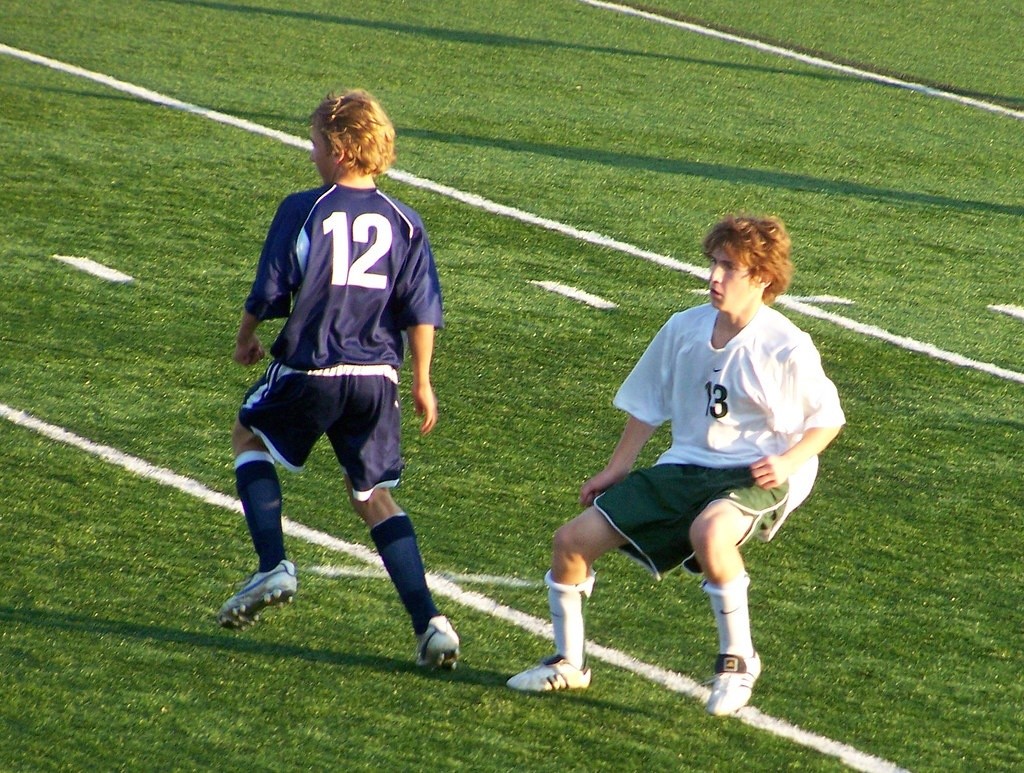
[
  {"xmin": 217, "ymin": 559, "xmax": 296, "ymax": 630},
  {"xmin": 505, "ymin": 654, "xmax": 592, "ymax": 692},
  {"xmin": 704, "ymin": 645, "xmax": 761, "ymax": 716},
  {"xmin": 416, "ymin": 615, "xmax": 461, "ymax": 671}
]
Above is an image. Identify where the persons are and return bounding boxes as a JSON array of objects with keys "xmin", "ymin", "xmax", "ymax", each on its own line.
[
  {"xmin": 214, "ymin": 88, "xmax": 461, "ymax": 673},
  {"xmin": 505, "ymin": 212, "xmax": 848, "ymax": 717}
]
